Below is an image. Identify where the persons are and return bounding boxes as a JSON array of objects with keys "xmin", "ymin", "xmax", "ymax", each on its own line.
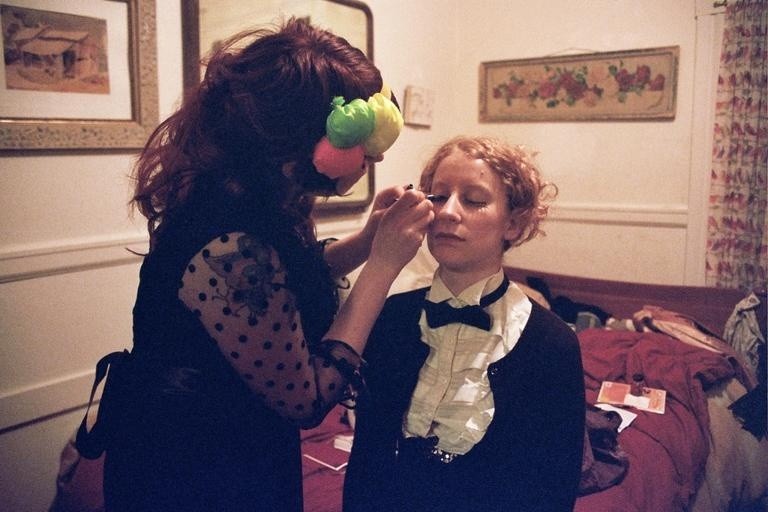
[
  {"xmin": 98, "ymin": 12, "xmax": 438, "ymax": 510},
  {"xmin": 328, "ymin": 135, "xmax": 589, "ymax": 511}
]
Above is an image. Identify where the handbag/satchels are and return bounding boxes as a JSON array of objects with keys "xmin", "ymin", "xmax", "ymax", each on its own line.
[{"xmin": 48, "ymin": 439, "xmax": 108, "ymax": 512}]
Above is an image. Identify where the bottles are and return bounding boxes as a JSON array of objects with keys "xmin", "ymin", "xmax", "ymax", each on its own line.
[{"xmin": 630, "ymin": 373, "xmax": 644, "ymax": 397}]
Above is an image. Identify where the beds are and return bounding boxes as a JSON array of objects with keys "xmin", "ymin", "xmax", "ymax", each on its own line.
[{"xmin": 54, "ymin": 263, "xmax": 768, "ymax": 512}]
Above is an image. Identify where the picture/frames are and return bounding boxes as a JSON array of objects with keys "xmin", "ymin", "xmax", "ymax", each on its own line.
[
  {"xmin": 476, "ymin": 45, "xmax": 679, "ymax": 123},
  {"xmin": 179, "ymin": 0, "xmax": 375, "ymax": 214},
  {"xmin": 0, "ymin": 0, "xmax": 161, "ymax": 151}
]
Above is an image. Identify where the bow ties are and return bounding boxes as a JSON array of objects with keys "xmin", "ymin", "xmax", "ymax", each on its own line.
[{"xmin": 423, "ymin": 298, "xmax": 490, "ymax": 331}]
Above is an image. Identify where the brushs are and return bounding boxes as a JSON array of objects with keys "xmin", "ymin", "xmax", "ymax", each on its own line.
[
  {"xmin": 405, "ymin": 184, "xmax": 414, "ymax": 190},
  {"xmin": 425, "ymin": 194, "xmax": 442, "ymax": 201}
]
